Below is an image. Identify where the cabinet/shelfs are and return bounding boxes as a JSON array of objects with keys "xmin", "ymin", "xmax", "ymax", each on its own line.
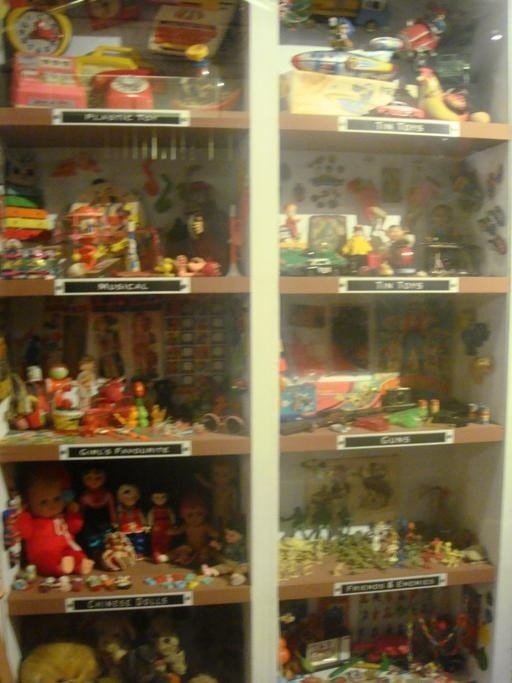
[
  {"xmin": 263, "ymin": 1, "xmax": 512, "ymax": 683},
  {"xmin": 0, "ymin": 0, "xmax": 264, "ymax": 683}
]
